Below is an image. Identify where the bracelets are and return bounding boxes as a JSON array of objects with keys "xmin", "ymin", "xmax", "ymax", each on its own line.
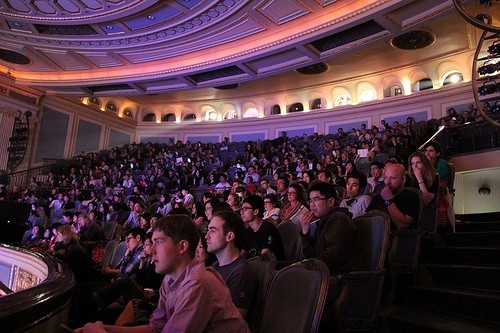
[{"xmin": 418, "ymin": 181, "xmax": 424, "ymax": 184}]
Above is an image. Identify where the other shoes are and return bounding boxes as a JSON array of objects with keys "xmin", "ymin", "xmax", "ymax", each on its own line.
[{"xmin": 76, "ymin": 286, "xmax": 101, "ymax": 315}]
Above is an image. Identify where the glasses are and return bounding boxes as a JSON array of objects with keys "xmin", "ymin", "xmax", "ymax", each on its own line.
[
  {"xmin": 240, "ymin": 207, "xmax": 253, "ymax": 212},
  {"xmin": 307, "ymin": 198, "xmax": 328, "ymax": 205}
]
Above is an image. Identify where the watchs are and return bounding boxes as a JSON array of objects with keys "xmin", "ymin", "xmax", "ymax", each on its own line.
[{"xmin": 385, "ymin": 199, "xmax": 394, "ymax": 206}]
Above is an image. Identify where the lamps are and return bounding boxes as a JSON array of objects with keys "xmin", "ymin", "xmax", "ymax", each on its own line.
[
  {"xmin": 477, "ymin": 78, "xmax": 500, "ymax": 96},
  {"xmin": 7, "ymin": 111, "xmax": 32, "ymax": 163},
  {"xmin": 478, "ymin": 61, "xmax": 500, "ymax": 75},
  {"xmin": 491, "ymin": 101, "xmax": 500, "ymax": 116},
  {"xmin": 487, "ymin": 40, "xmax": 500, "ymax": 53}
]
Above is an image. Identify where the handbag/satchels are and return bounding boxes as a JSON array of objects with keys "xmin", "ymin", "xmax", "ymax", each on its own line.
[{"xmin": 439, "ymin": 190, "xmax": 457, "ymax": 226}]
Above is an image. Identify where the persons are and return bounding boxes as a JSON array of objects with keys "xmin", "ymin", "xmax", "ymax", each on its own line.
[
  {"xmin": 74, "ymin": 213, "xmax": 251, "ymax": 333},
  {"xmin": 0, "ymin": 100, "xmax": 500, "ymax": 328}
]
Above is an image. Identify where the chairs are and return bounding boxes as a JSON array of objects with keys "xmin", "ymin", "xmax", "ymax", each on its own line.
[{"xmin": 81, "ymin": 125, "xmax": 500, "ymax": 333}]
